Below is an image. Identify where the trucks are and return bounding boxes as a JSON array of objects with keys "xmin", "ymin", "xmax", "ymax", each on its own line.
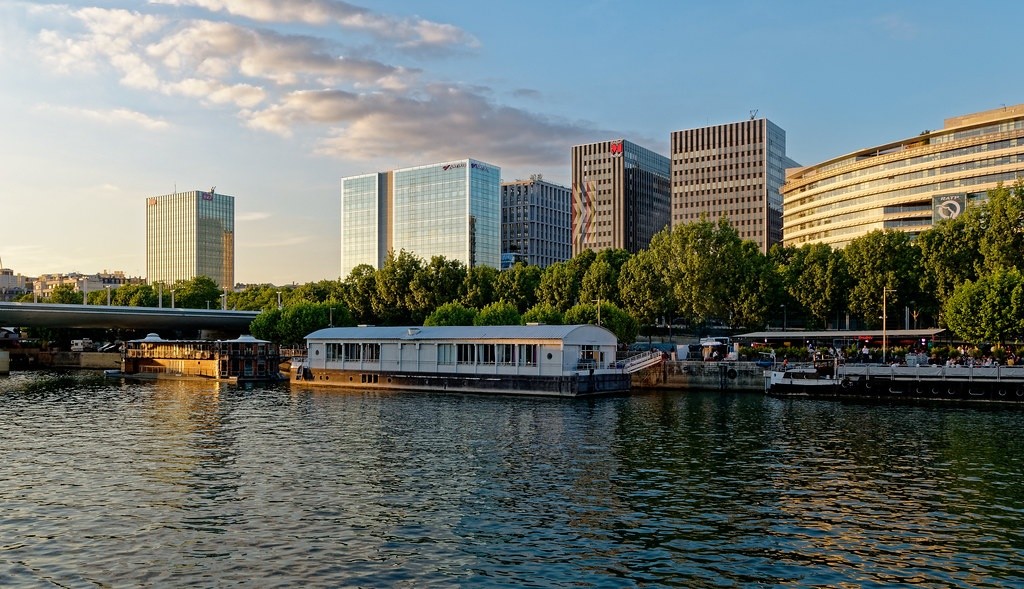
[{"xmin": 71, "ymin": 338, "xmax": 93, "ymax": 352}]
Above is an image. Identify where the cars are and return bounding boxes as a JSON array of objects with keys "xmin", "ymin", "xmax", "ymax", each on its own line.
[{"xmin": 98, "ymin": 343, "xmax": 132, "ymax": 353}]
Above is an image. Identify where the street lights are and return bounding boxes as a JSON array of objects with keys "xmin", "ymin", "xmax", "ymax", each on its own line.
[
  {"xmin": 591, "ymin": 300, "xmax": 600, "ymax": 326},
  {"xmin": 220, "ymin": 286, "xmax": 228, "ymax": 310},
  {"xmin": 878, "ymin": 290, "xmax": 898, "ymax": 367},
  {"xmin": 276, "ymin": 292, "xmax": 282, "ymax": 304},
  {"xmin": 106, "ymin": 285, "xmax": 111, "ymax": 306},
  {"xmin": 158, "ymin": 282, "xmax": 175, "ymax": 308}
]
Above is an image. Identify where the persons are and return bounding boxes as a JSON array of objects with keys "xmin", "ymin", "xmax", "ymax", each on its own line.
[{"xmin": 617, "ymin": 340, "xmax": 1024, "ymax": 372}]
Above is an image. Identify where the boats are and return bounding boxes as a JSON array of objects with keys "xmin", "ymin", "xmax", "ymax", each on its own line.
[
  {"xmin": 290, "ymin": 323, "xmax": 633, "ymax": 397},
  {"xmin": 104, "ymin": 333, "xmax": 289, "ymax": 383}
]
